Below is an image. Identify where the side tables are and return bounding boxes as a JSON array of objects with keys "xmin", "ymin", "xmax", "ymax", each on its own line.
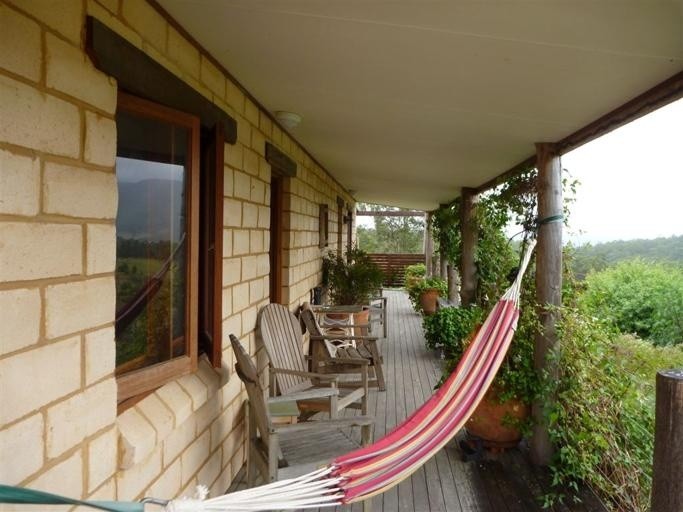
[
  {"xmin": 313, "ymin": 305, "xmax": 363, "ymax": 347},
  {"xmin": 246, "ymin": 398, "xmax": 300, "ymax": 477}
]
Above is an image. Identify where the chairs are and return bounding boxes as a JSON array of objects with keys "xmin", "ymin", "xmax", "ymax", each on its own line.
[
  {"xmin": 230, "ymin": 334, "xmax": 374, "ymax": 512},
  {"xmin": 301, "ymin": 302, "xmax": 387, "ymax": 391},
  {"xmin": 258, "ymin": 303, "xmax": 370, "ymax": 419},
  {"xmin": 369, "ymin": 288, "xmax": 387, "ymax": 339}
]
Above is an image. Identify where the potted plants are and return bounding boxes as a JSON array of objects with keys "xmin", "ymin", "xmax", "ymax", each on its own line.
[
  {"xmin": 405, "ymin": 262, "xmax": 427, "ymax": 291},
  {"xmin": 323, "ymin": 245, "xmax": 385, "ymax": 341},
  {"xmin": 467, "ymin": 304, "xmax": 610, "ymax": 510},
  {"xmin": 409, "ymin": 275, "xmax": 448, "ymax": 314},
  {"xmin": 422, "ymin": 306, "xmax": 482, "ymax": 358}
]
